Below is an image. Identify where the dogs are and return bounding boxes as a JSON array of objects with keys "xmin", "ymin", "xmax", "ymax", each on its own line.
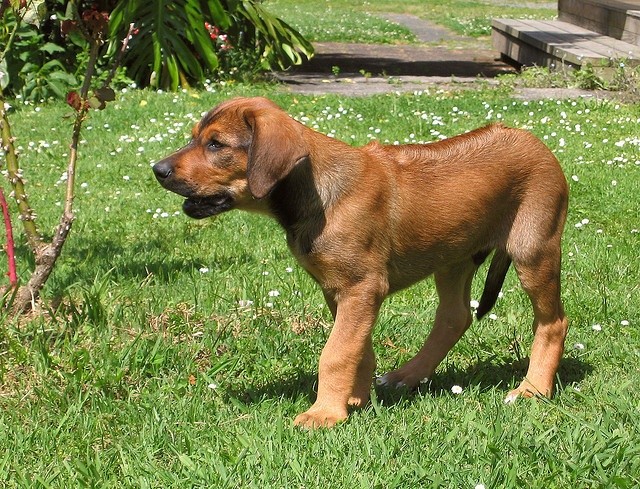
[{"xmin": 152, "ymin": 95, "xmax": 570, "ymax": 434}]
